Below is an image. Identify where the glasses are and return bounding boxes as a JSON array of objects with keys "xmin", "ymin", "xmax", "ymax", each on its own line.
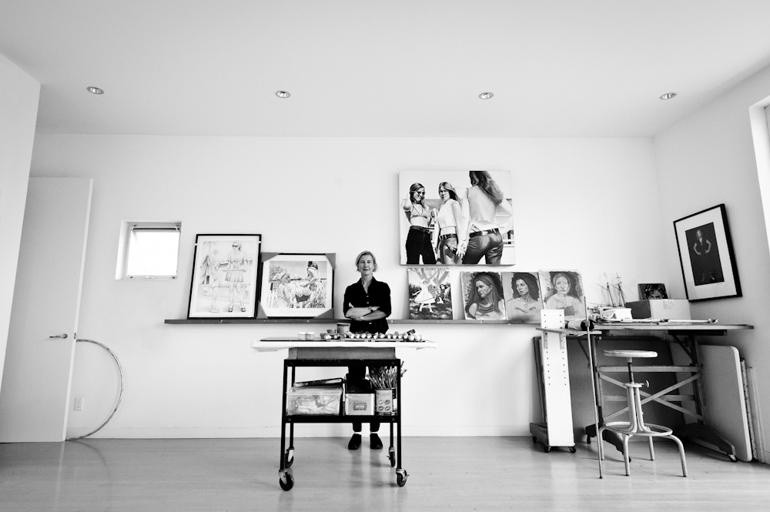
[{"xmin": 416, "ymin": 190, "xmax": 425, "ymax": 195}]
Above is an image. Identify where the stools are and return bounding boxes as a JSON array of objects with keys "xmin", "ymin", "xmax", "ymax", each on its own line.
[{"xmin": 599, "ymin": 349, "xmax": 687, "ymax": 477}]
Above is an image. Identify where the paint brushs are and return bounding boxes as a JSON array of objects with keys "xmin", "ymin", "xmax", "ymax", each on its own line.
[{"xmin": 367, "ymin": 361, "xmax": 406, "ymax": 390}]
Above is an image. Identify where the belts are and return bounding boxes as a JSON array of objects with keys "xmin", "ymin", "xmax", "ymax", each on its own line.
[
  {"xmin": 410, "ymin": 226, "xmax": 429, "ymax": 233},
  {"xmin": 470, "ymin": 228, "xmax": 498, "ymax": 237},
  {"xmin": 438, "ymin": 234, "xmax": 457, "ymax": 241}
]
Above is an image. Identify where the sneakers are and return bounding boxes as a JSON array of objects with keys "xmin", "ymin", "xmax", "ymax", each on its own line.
[
  {"xmin": 370, "ymin": 433, "xmax": 382, "ymax": 449},
  {"xmin": 348, "ymin": 434, "xmax": 361, "ymax": 449}
]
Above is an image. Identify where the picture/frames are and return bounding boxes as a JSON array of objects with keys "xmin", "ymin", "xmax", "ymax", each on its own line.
[
  {"xmin": 187, "ymin": 233, "xmax": 336, "ymax": 321},
  {"xmin": 673, "ymin": 203, "xmax": 742, "ymax": 303}
]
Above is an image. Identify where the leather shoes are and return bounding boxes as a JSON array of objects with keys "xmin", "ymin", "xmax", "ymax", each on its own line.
[
  {"xmin": 241, "ymin": 304, "xmax": 246, "ymax": 312},
  {"xmin": 228, "ymin": 303, "xmax": 233, "ymax": 311}
]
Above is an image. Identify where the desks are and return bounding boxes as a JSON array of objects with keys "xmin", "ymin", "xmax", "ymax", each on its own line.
[
  {"xmin": 260, "ymin": 339, "xmax": 426, "ymax": 494},
  {"xmin": 585, "ymin": 323, "xmax": 754, "ymax": 464}
]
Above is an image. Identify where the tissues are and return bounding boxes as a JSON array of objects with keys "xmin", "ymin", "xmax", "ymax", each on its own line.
[{"xmin": 599, "ymin": 305, "xmax": 635, "ymax": 322}]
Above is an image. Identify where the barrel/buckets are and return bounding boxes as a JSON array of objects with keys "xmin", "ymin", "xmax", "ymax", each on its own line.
[{"xmin": 337, "ymin": 323, "xmax": 352, "ymax": 337}]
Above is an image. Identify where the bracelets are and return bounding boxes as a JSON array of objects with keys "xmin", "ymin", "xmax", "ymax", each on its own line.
[{"xmin": 369, "ymin": 306, "xmax": 373, "ymax": 313}]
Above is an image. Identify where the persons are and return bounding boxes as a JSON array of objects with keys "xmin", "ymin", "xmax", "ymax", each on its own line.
[
  {"xmin": 505, "ymin": 272, "xmax": 542, "ymax": 320},
  {"xmin": 225, "ymin": 240, "xmax": 250, "ymax": 312},
  {"xmin": 342, "ymin": 249, "xmax": 392, "ymax": 450},
  {"xmin": 459, "ymin": 169, "xmax": 513, "ymax": 265},
  {"xmin": 545, "ymin": 271, "xmax": 583, "ymax": 315},
  {"xmin": 414, "ymin": 279, "xmax": 434, "ymax": 313},
  {"xmin": 464, "ymin": 272, "xmax": 503, "ymax": 318},
  {"xmin": 691, "ymin": 229, "xmax": 716, "ymax": 283},
  {"xmin": 403, "ymin": 182, "xmax": 437, "ymax": 265},
  {"xmin": 432, "ymin": 180, "xmax": 464, "ymax": 265}
]
